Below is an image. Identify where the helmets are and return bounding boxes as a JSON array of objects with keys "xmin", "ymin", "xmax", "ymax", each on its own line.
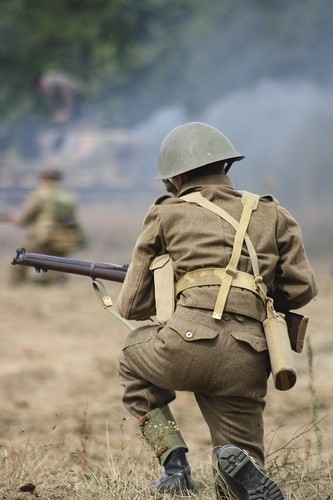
[{"xmin": 157, "ymin": 122, "xmax": 245, "ymax": 181}]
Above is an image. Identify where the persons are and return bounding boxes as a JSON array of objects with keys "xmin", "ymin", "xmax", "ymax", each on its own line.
[
  {"xmin": 116, "ymin": 121, "xmax": 319, "ymax": 500},
  {"xmin": 1, "ymin": 163, "xmax": 87, "ymax": 287}
]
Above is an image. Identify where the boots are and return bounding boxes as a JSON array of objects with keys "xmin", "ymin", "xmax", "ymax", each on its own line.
[
  {"xmin": 216, "ymin": 445, "xmax": 284, "ymax": 500},
  {"xmin": 145, "ymin": 447, "xmax": 195, "ymax": 495}
]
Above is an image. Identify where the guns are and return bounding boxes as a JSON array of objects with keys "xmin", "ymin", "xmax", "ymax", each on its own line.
[{"xmin": 11, "ymin": 247, "xmax": 307, "ymax": 356}]
